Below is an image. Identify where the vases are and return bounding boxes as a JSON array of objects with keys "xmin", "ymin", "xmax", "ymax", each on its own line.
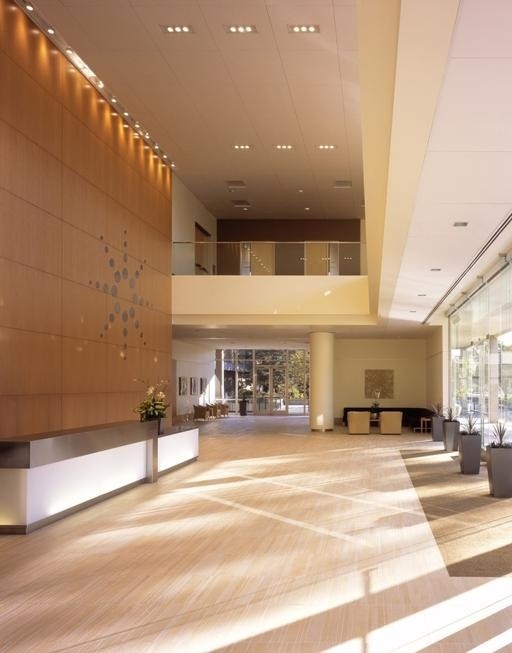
[{"xmin": 149, "ymin": 417, "xmax": 165, "ymax": 435}]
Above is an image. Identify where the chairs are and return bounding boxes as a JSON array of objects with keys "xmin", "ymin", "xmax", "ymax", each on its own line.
[
  {"xmin": 193, "ymin": 402, "xmax": 229, "ymax": 421},
  {"xmin": 346, "ymin": 409, "xmax": 403, "ymax": 434}
]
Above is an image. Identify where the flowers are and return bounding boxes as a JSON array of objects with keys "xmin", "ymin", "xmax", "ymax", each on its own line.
[{"xmin": 132, "ymin": 376, "xmax": 171, "ymax": 423}]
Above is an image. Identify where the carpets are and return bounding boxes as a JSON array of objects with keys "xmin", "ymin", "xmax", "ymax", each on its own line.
[{"xmin": 400, "ymin": 449, "xmax": 512, "ymax": 577}]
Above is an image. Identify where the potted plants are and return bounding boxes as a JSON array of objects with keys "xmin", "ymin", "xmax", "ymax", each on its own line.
[
  {"xmin": 237, "ymin": 369, "xmax": 249, "ymax": 416},
  {"xmin": 430, "ymin": 402, "xmax": 512, "ymax": 498},
  {"xmin": 370, "ymin": 401, "xmax": 379, "ymax": 418}
]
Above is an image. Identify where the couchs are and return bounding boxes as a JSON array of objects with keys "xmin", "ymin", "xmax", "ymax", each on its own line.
[{"xmin": 343, "ymin": 407, "xmax": 434, "ymax": 435}]
